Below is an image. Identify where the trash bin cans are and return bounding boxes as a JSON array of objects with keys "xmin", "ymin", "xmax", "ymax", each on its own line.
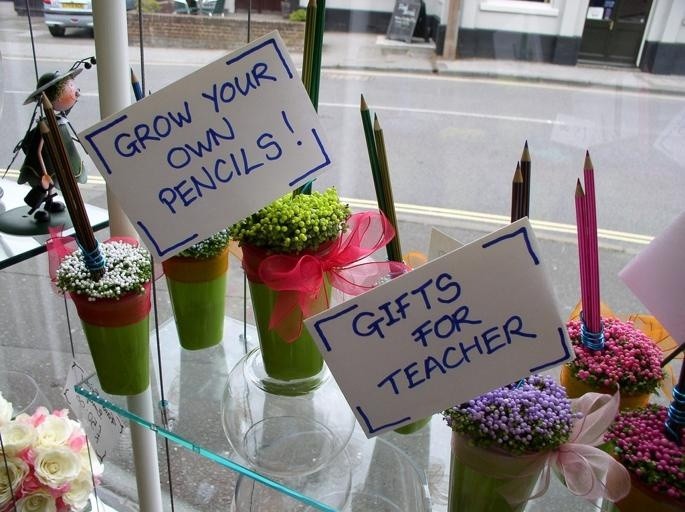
[{"xmin": 131, "ymin": 67, "xmax": 151, "ymax": 100}]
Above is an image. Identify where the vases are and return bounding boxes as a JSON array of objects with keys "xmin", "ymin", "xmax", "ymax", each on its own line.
[
  {"xmin": 448, "ymin": 430, "xmax": 548, "ymax": 512},
  {"xmin": 608, "ymin": 460, "xmax": 684, "ymax": 511}
]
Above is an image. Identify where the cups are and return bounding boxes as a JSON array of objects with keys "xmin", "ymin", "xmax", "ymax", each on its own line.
[
  {"xmin": 303, "ymin": 488, "xmax": 399, "ymax": 511},
  {"xmin": 1, "ymin": 368, "xmax": 53, "ymax": 419}
]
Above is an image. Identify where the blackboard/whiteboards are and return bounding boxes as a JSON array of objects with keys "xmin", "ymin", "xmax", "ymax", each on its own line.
[{"xmin": 387, "ymin": 0, "xmax": 422, "ymax": 40}]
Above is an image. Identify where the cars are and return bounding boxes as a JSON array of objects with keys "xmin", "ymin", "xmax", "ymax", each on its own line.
[{"xmin": 42, "ymin": 0, "xmax": 138, "ymax": 37}]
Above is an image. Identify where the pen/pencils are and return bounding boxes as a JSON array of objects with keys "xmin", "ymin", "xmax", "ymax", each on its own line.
[
  {"xmin": 511, "ymin": 141, "xmax": 531, "ymax": 221},
  {"xmin": 575, "ymin": 150, "xmax": 601, "ymax": 331},
  {"xmin": 358, "ymin": 93, "xmax": 404, "ymax": 278},
  {"xmin": 37, "ymin": 91, "xmax": 108, "ymax": 281}
]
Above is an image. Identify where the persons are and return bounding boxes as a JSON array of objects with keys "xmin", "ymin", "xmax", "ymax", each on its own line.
[{"xmin": 17, "ymin": 69, "xmax": 86, "ymax": 220}]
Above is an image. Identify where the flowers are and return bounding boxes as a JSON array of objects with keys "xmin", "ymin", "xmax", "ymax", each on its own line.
[
  {"xmin": 0, "ymin": 392, "xmax": 104, "ymax": 512},
  {"xmin": 175, "ymin": 187, "xmax": 354, "ymax": 261},
  {"xmin": 54, "ymin": 238, "xmax": 156, "ymax": 304}
]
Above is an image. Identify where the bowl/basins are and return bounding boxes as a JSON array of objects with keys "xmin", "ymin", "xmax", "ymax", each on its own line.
[{"xmin": 226, "ymin": 427, "xmax": 433, "ymax": 511}]
